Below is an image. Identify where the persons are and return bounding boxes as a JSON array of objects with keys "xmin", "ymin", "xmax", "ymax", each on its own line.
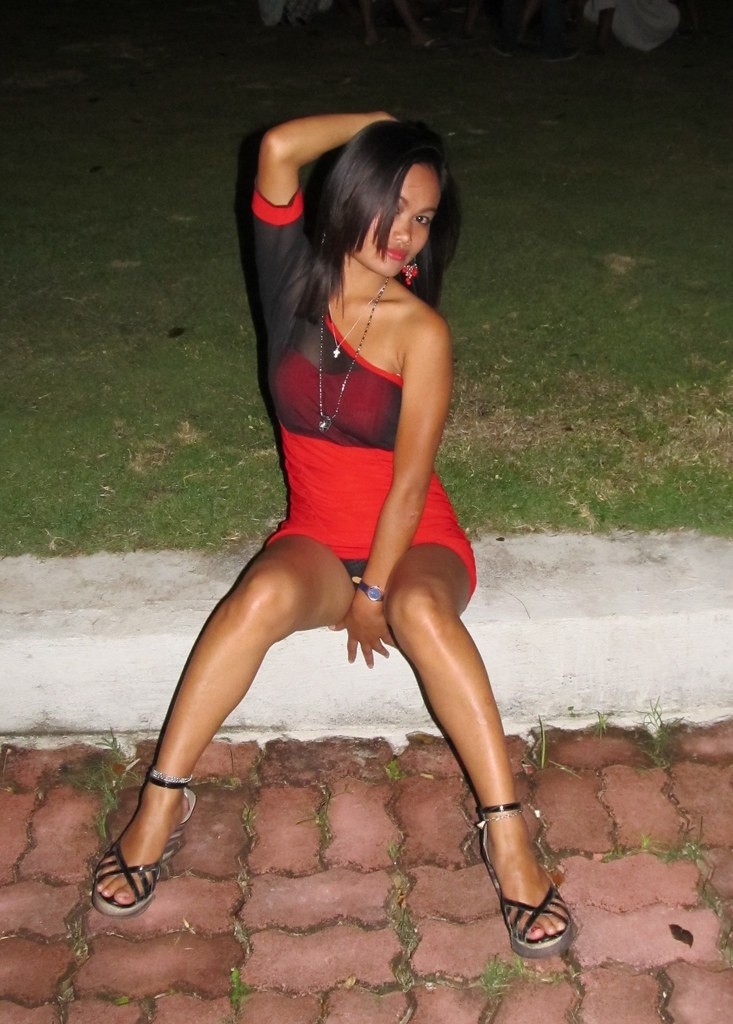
[{"xmin": 90, "ymin": 108, "xmax": 579, "ymax": 957}]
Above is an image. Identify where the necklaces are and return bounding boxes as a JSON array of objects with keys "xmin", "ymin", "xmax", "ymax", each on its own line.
[
  {"xmin": 328, "ymin": 283, "xmax": 386, "ymax": 359},
  {"xmin": 317, "ymin": 276, "xmax": 390, "ymax": 433}
]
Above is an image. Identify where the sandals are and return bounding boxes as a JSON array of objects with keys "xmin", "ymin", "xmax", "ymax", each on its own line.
[
  {"xmin": 90, "ymin": 769, "xmax": 197, "ymax": 917},
  {"xmin": 474, "ymin": 800, "xmax": 576, "ymax": 958}
]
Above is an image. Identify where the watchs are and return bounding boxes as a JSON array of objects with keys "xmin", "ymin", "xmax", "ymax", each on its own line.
[{"xmin": 352, "ymin": 576, "xmax": 388, "ymax": 602}]
